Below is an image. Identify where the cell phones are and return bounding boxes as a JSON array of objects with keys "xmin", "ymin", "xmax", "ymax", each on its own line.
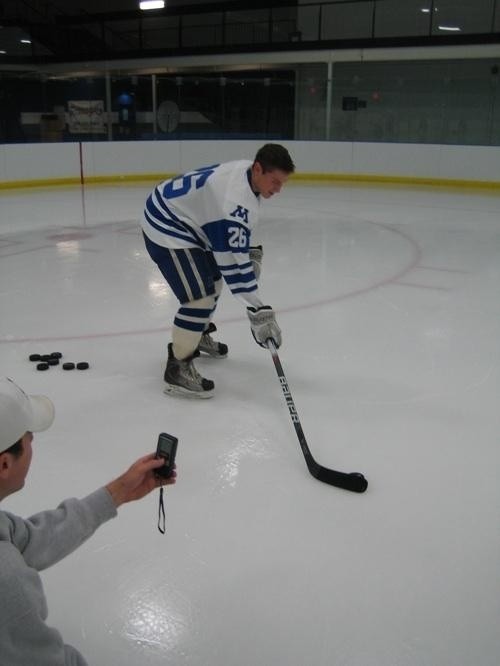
[{"xmin": 152, "ymin": 433, "xmax": 178, "ymax": 480}]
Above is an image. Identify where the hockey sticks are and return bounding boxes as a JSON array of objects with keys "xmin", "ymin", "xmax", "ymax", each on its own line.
[{"xmin": 266, "ymin": 338, "xmax": 368, "ymax": 494}]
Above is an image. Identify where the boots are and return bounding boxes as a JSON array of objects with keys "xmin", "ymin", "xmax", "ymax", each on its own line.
[
  {"xmin": 196, "ymin": 322, "xmax": 228, "ymax": 355},
  {"xmin": 163, "ymin": 342, "xmax": 215, "ymax": 393}
]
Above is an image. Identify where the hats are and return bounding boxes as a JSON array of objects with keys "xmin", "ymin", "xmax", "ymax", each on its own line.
[{"xmin": 0, "ymin": 374, "xmax": 55, "ymax": 454}]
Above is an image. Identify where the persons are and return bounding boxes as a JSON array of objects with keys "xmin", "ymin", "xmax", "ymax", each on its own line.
[
  {"xmin": 1, "ymin": 372, "xmax": 178, "ymax": 666},
  {"xmin": 140, "ymin": 144, "xmax": 295, "ymax": 391}
]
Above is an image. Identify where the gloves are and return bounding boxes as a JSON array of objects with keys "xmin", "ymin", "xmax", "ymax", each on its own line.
[
  {"xmin": 246, "ymin": 305, "xmax": 283, "ymax": 349},
  {"xmin": 247, "ymin": 244, "xmax": 264, "ymax": 282}
]
